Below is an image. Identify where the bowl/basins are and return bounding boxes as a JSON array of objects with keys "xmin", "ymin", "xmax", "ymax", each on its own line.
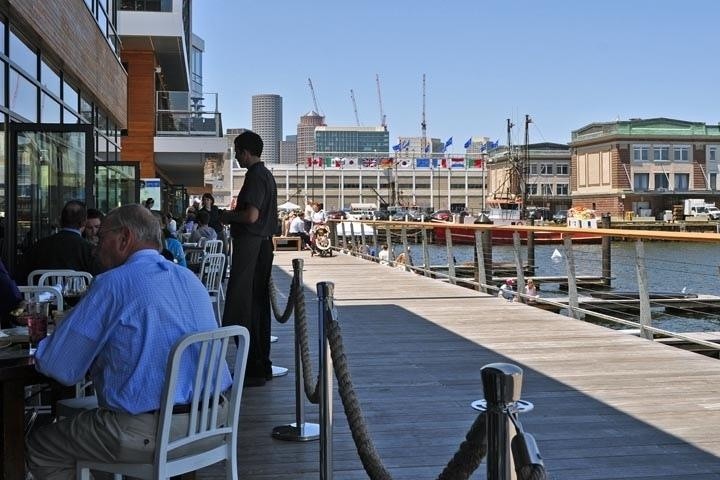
[
  {"xmin": 1, "ymin": 310, "xmax": 48, "ymax": 350},
  {"xmin": 184, "ymin": 241, "xmax": 199, "ymax": 250}
]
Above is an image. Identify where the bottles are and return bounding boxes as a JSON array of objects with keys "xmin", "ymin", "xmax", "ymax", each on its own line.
[
  {"xmin": 173, "ymin": 258, "xmax": 179, "ymax": 266},
  {"xmin": 17, "ymin": 149, "xmax": 50, "ymax": 253},
  {"xmin": 231, "ymin": 194, "xmax": 240, "ymax": 211},
  {"xmin": 199, "ymin": 236, "xmax": 207, "ymax": 249}
]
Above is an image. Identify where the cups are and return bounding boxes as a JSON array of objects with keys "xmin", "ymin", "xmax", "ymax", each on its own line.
[
  {"xmin": 183, "ymin": 232, "xmax": 191, "ymax": 242},
  {"xmin": 54, "ymin": 315, "xmax": 65, "ymax": 328},
  {"xmin": 26, "ymin": 302, "xmax": 49, "ymax": 347},
  {"xmin": 54, "ymin": 276, "xmax": 88, "ymax": 297}
]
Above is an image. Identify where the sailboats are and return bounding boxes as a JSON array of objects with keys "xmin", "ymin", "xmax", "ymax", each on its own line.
[{"xmin": 431, "ymin": 114, "xmax": 602, "ymax": 245}]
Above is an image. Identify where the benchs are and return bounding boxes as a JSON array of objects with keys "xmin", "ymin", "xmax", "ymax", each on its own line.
[{"xmin": 273, "ymin": 236, "xmax": 303, "ymax": 252}]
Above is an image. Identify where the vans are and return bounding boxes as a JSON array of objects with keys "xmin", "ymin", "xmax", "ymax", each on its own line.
[{"xmin": 521, "ymin": 209, "xmax": 553, "ymax": 221}]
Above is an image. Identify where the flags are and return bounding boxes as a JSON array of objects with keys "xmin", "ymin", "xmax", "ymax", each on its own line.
[{"xmin": 305, "ymin": 136, "xmax": 499, "ymax": 169}]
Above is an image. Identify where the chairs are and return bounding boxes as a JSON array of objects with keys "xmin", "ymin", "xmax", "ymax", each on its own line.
[
  {"xmin": 74, "ymin": 326, "xmax": 250, "ymax": 480},
  {"xmin": 2, "ymin": 234, "xmax": 253, "ymax": 388}
]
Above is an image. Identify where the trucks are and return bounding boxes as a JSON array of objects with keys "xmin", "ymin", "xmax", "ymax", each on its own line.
[{"xmin": 679, "ymin": 198, "xmax": 720, "ymax": 221}]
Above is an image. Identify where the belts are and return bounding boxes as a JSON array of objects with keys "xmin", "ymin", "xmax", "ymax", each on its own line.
[{"xmin": 146, "ymin": 393, "xmax": 224, "ymax": 414}]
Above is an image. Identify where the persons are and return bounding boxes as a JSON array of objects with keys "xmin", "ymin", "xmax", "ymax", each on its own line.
[
  {"xmin": 30, "ymin": 202, "xmax": 231, "ymax": 478},
  {"xmin": 0, "ymin": 192, "xmax": 230, "ymax": 341},
  {"xmin": 281, "ymin": 200, "xmax": 412, "ymax": 267},
  {"xmin": 498, "ymin": 278, "xmax": 515, "ymax": 299},
  {"xmin": 524, "ymin": 279, "xmax": 538, "ymax": 297},
  {"xmin": 210, "ymin": 129, "xmax": 281, "ymax": 390}
]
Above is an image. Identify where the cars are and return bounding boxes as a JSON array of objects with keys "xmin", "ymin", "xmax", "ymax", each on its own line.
[{"xmin": 552, "ymin": 209, "xmax": 570, "ymax": 224}]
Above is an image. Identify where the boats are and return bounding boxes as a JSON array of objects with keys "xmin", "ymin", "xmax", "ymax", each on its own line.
[{"xmin": 333, "ymin": 203, "xmax": 453, "ymax": 246}]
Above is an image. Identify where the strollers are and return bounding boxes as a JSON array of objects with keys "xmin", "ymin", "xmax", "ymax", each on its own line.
[{"xmin": 310, "ymin": 220, "xmax": 333, "ymax": 258}]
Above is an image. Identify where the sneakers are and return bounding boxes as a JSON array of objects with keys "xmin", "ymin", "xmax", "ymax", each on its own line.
[{"xmin": 232, "ymin": 371, "xmax": 272, "ymax": 386}]
